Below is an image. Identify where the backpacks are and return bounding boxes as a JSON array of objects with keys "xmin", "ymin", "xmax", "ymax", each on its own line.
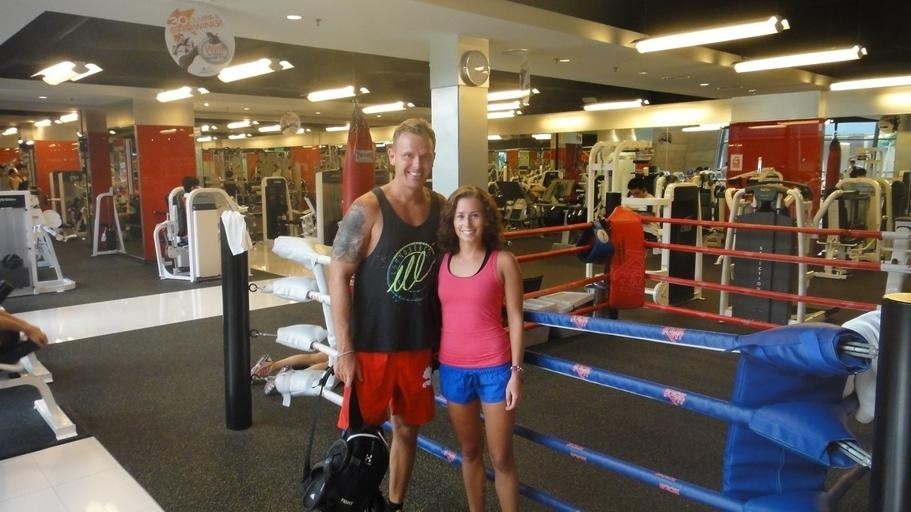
[{"xmin": 302, "ymin": 366, "xmax": 389, "ymax": 512}]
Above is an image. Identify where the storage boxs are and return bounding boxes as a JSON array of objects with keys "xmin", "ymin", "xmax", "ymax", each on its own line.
[
  {"xmin": 522, "ymin": 297, "xmax": 556, "ymax": 349},
  {"xmin": 538, "ymin": 290, "xmax": 593, "ymax": 363}
]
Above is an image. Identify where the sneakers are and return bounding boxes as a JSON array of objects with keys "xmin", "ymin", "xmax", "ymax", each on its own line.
[
  {"xmin": 264, "ymin": 365, "xmax": 290, "ymax": 395},
  {"xmin": 250, "ymin": 354, "xmax": 273, "ymax": 383}
]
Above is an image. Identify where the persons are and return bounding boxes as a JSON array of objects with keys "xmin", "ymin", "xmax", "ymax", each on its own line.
[
  {"xmin": 847, "ymin": 160, "xmax": 862, "ymax": 178},
  {"xmin": 326, "ymin": 117, "xmax": 507, "ymax": 512},
  {"xmin": 251, "ymin": 349, "xmax": 330, "ymax": 396},
  {"xmin": 182, "ymin": 176, "xmax": 202, "ymax": 234},
  {"xmin": 0, "ymin": 304, "xmax": 48, "ymax": 379},
  {"xmin": 625, "ymin": 177, "xmax": 659, "ymax": 249},
  {"xmin": 7, "ymin": 169, "xmax": 23, "ymax": 190},
  {"xmin": 437, "ymin": 184, "xmax": 526, "ymax": 512},
  {"xmin": 685, "ymin": 161, "xmax": 728, "ymax": 183},
  {"xmin": 223, "ymin": 170, "xmax": 242, "ymax": 203}
]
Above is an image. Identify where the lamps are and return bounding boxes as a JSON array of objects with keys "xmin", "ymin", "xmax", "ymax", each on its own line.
[
  {"xmin": 730, "ymin": 1, "xmax": 868, "ymax": 74},
  {"xmin": 578, "ymin": 98, "xmax": 650, "ymax": 111},
  {"xmin": 359, "ymin": 98, "xmax": 415, "ymax": 116},
  {"xmin": 155, "ymin": 64, "xmax": 210, "ymax": 103},
  {"xmin": 217, "ymin": 42, "xmax": 294, "ymax": 84},
  {"xmin": 630, "ymin": 1, "xmax": 791, "ymax": 55},
  {"xmin": 298, "ymin": 52, "xmax": 369, "ymax": 103},
  {"xmin": 487, "ymin": 80, "xmax": 541, "ymax": 119},
  {"xmin": 29, "ymin": 36, "xmax": 103, "ymax": 87}
]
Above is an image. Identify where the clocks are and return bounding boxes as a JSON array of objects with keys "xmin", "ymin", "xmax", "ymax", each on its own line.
[{"xmin": 461, "ymin": 50, "xmax": 490, "ymax": 87}]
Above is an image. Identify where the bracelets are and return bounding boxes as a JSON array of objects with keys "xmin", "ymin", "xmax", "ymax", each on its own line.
[
  {"xmin": 510, "ymin": 364, "xmax": 525, "ymax": 378},
  {"xmin": 334, "ymin": 350, "xmax": 355, "ymax": 360}
]
